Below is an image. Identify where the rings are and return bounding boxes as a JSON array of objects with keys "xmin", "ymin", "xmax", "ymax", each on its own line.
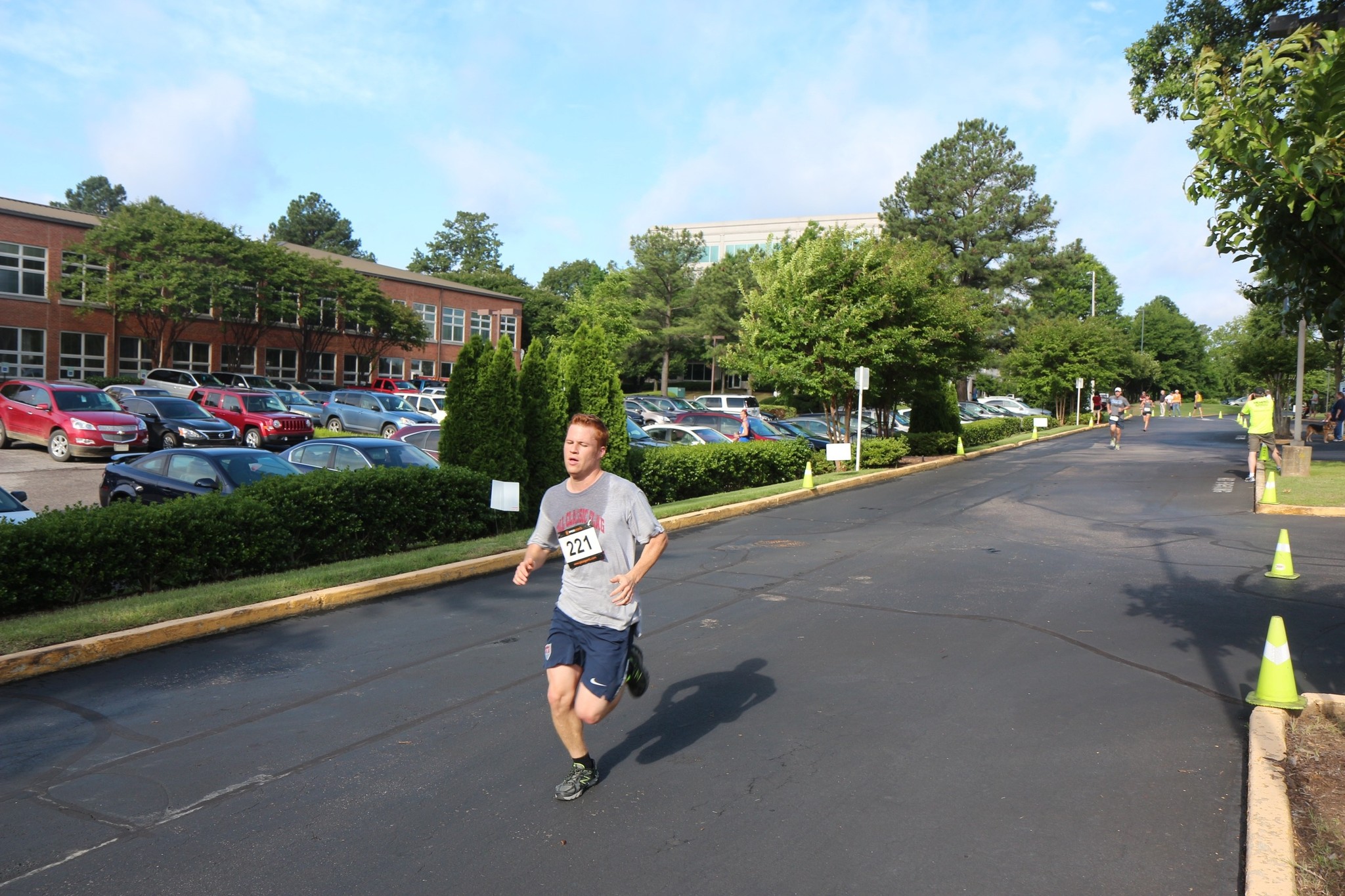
[{"xmin": 626, "ymin": 595, "xmax": 630, "ymax": 599}]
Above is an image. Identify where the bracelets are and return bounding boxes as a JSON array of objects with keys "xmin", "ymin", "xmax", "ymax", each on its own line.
[{"xmin": 739, "ymin": 435, "xmax": 740, "ymax": 437}]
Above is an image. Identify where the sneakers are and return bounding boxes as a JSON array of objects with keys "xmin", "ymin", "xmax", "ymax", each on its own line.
[
  {"xmin": 626, "ymin": 644, "xmax": 650, "ymax": 698},
  {"xmin": 1244, "ymin": 476, "xmax": 1255, "ymax": 482},
  {"xmin": 1276, "ymin": 466, "xmax": 1281, "ymax": 471},
  {"xmin": 555, "ymin": 759, "xmax": 600, "ymax": 801}
]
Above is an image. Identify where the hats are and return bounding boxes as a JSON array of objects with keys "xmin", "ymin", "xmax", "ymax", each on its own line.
[
  {"xmin": 1114, "ymin": 387, "xmax": 1122, "ymax": 392},
  {"xmin": 1251, "ymin": 387, "xmax": 1265, "ymax": 394}
]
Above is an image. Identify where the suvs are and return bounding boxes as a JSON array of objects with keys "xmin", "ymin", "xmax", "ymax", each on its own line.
[{"xmin": 0, "ymin": 378, "xmax": 149, "ymax": 463}]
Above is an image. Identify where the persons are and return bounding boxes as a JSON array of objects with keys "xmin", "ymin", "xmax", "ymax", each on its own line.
[
  {"xmin": 1190, "ymin": 391, "xmax": 1203, "ymax": 418},
  {"xmin": 1093, "ymin": 391, "xmax": 1101, "ymax": 424},
  {"xmin": 1331, "ymin": 392, "xmax": 1345, "ymax": 442},
  {"xmin": 1240, "ymin": 387, "xmax": 1282, "ymax": 482},
  {"xmin": 1107, "ymin": 387, "xmax": 1131, "ymax": 450},
  {"xmin": 1307, "ymin": 390, "xmax": 1318, "ymax": 419},
  {"xmin": 1139, "ymin": 391, "xmax": 1155, "ymax": 431},
  {"xmin": 733, "ymin": 411, "xmax": 751, "ymax": 443},
  {"xmin": 1159, "ymin": 389, "xmax": 1181, "ymax": 417},
  {"xmin": 513, "ymin": 414, "xmax": 669, "ymax": 801}
]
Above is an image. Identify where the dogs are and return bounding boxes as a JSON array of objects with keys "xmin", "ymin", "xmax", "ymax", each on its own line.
[{"xmin": 1305, "ymin": 420, "xmax": 1338, "ymax": 443}]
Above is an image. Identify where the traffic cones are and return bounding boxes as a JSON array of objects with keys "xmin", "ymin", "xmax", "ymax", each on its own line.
[
  {"xmin": 1189, "ymin": 412, "xmax": 1193, "ymax": 418},
  {"xmin": 1235, "ymin": 413, "xmax": 1241, "ymax": 421},
  {"xmin": 1088, "ymin": 416, "xmax": 1094, "ymax": 427},
  {"xmin": 1241, "ymin": 418, "xmax": 1249, "ymax": 429},
  {"xmin": 1257, "ymin": 442, "xmax": 1271, "ymax": 461},
  {"xmin": 1264, "ymin": 528, "xmax": 1300, "ymax": 579},
  {"xmin": 952, "ymin": 436, "xmax": 966, "ymax": 456},
  {"xmin": 1218, "ymin": 411, "xmax": 1224, "ymax": 419},
  {"xmin": 1245, "ymin": 615, "xmax": 1308, "ymax": 711},
  {"xmin": 1151, "ymin": 409, "xmax": 1154, "ymax": 417},
  {"xmin": 1257, "ymin": 471, "xmax": 1281, "ymax": 505},
  {"xmin": 1030, "ymin": 426, "xmax": 1039, "ymax": 440},
  {"xmin": 800, "ymin": 461, "xmax": 817, "ymax": 490},
  {"xmin": 1238, "ymin": 416, "xmax": 1243, "ymax": 425}
]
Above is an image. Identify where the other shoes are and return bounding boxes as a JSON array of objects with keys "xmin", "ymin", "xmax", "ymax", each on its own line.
[
  {"xmin": 1110, "ymin": 438, "xmax": 1115, "ymax": 447},
  {"xmin": 1332, "ymin": 439, "xmax": 1343, "ymax": 442},
  {"xmin": 1159, "ymin": 414, "xmax": 1164, "ymax": 417},
  {"xmin": 1114, "ymin": 444, "xmax": 1120, "ymax": 450},
  {"xmin": 1143, "ymin": 429, "xmax": 1146, "ymax": 432},
  {"xmin": 1096, "ymin": 421, "xmax": 1099, "ymax": 425}
]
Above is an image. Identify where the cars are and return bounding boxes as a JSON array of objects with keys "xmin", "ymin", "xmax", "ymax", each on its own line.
[
  {"xmin": 958, "ymin": 393, "xmax": 1052, "ymax": 425},
  {"xmin": 250, "ymin": 437, "xmax": 441, "ymax": 481},
  {"xmin": 99, "ymin": 445, "xmax": 308, "ymax": 507},
  {"xmin": 0, "ymin": 483, "xmax": 37, "ymax": 528},
  {"xmin": 146, "ymin": 367, "xmax": 450, "ymax": 463},
  {"xmin": 623, "ymin": 393, "xmax": 913, "ymax": 456},
  {"xmin": 1228, "ymin": 397, "xmax": 1248, "ymax": 406},
  {"xmin": 117, "ymin": 396, "xmax": 242, "ymax": 453},
  {"xmin": 100, "ymin": 384, "xmax": 173, "ymax": 407},
  {"xmin": 1220, "ymin": 398, "xmax": 1239, "ymax": 405}
]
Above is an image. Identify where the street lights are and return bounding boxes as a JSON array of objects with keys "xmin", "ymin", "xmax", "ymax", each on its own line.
[
  {"xmin": 1135, "ymin": 309, "xmax": 1145, "ymax": 354},
  {"xmin": 1086, "ymin": 270, "xmax": 1096, "ymax": 318},
  {"xmin": 1324, "ymin": 366, "xmax": 1336, "ymax": 413}
]
